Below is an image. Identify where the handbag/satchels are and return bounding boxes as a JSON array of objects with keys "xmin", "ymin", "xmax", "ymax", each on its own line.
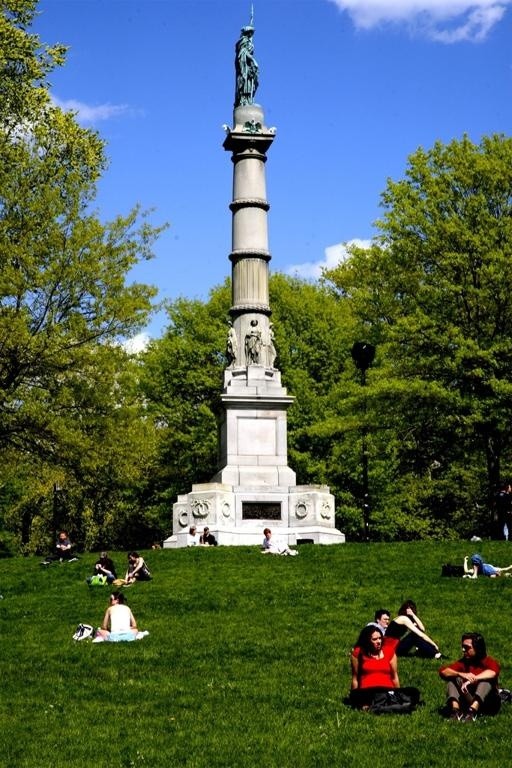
[{"xmin": 73, "ymin": 623, "xmax": 95, "ymax": 642}]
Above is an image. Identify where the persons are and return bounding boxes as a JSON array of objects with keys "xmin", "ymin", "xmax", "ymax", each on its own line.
[
  {"xmin": 200, "ymin": 526, "xmax": 217, "ymax": 547},
  {"xmin": 86, "ymin": 551, "xmax": 118, "ymax": 584},
  {"xmin": 151, "ymin": 541, "xmax": 162, "ymax": 550},
  {"xmin": 120, "ymin": 551, "xmax": 153, "ymax": 588},
  {"xmin": 234, "ymin": 25, "xmax": 260, "ymax": 106},
  {"xmin": 245, "ymin": 319, "xmax": 263, "ymax": 365},
  {"xmin": 267, "ymin": 322, "xmax": 277, "ymax": 368},
  {"xmin": 262, "ymin": 528, "xmax": 299, "ymax": 556},
  {"xmin": 38, "ymin": 530, "xmax": 79, "ymax": 566},
  {"xmin": 348, "ymin": 599, "xmax": 450, "ymax": 714},
  {"xmin": 187, "ymin": 525, "xmax": 200, "ymax": 547},
  {"xmin": 225, "ymin": 320, "xmax": 238, "ymax": 370},
  {"xmin": 95, "ymin": 591, "xmax": 139, "ymax": 642},
  {"xmin": 496, "ymin": 484, "xmax": 512, "ymax": 541},
  {"xmin": 462, "ymin": 553, "xmax": 512, "ymax": 579},
  {"xmin": 438, "ymin": 632, "xmax": 502, "ymax": 723}
]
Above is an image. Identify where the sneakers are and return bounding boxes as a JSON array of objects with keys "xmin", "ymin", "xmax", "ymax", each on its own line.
[{"xmin": 443, "ymin": 705, "xmax": 479, "ymax": 724}]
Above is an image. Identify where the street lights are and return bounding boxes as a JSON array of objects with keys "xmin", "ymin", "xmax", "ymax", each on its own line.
[{"xmin": 352, "ymin": 342, "xmax": 375, "ymax": 539}]
